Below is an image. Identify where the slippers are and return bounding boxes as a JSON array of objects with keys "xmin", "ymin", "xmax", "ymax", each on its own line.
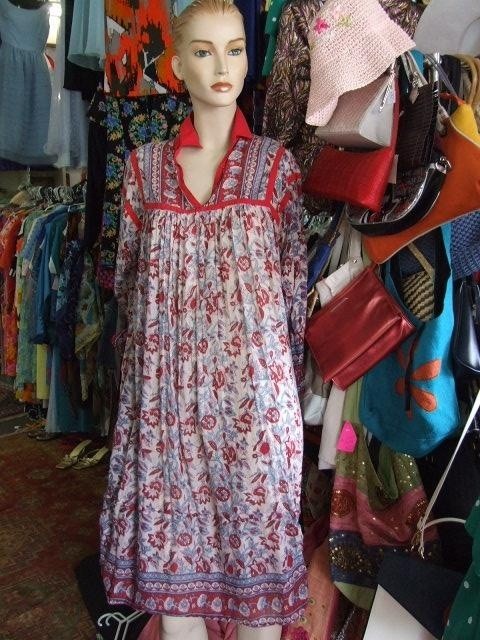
[
  {"xmin": 71, "ymin": 445, "xmax": 110, "ymax": 470},
  {"xmin": 54, "ymin": 439, "xmax": 92, "ymax": 470}
]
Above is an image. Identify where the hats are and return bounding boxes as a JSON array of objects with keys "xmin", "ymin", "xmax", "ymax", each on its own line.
[{"xmin": 304, "ymin": 0, "xmax": 418, "ymax": 127}]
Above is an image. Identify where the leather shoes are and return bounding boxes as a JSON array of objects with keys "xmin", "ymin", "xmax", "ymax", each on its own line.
[
  {"xmin": 27, "ymin": 426, "xmax": 49, "ymax": 437},
  {"xmin": 35, "ymin": 431, "xmax": 60, "ymax": 440}
]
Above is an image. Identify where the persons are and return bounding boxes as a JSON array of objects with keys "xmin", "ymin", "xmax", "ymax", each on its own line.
[{"xmin": 98, "ymin": 1, "xmax": 309, "ymax": 640}]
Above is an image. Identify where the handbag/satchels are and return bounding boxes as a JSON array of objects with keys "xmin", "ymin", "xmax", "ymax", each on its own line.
[{"xmin": 303, "ymin": 49, "xmax": 480, "ymax": 640}]
